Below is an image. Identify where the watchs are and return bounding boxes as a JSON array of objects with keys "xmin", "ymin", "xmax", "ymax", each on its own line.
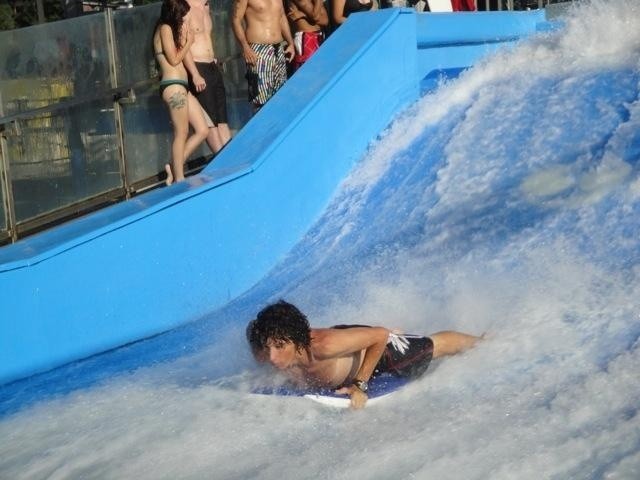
[{"xmin": 353, "ymin": 377, "xmax": 370, "ymax": 392}]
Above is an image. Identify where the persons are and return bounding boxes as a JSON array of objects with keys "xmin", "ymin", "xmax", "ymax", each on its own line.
[
  {"xmin": 245, "ymin": 301, "xmax": 493, "ymax": 410},
  {"xmin": 232, "ymin": 0, "xmax": 380, "ymax": 111},
  {"xmin": 179, "ymin": 1, "xmax": 233, "ymax": 157},
  {"xmin": 151, "ymin": 1, "xmax": 210, "ymax": 187}
]
